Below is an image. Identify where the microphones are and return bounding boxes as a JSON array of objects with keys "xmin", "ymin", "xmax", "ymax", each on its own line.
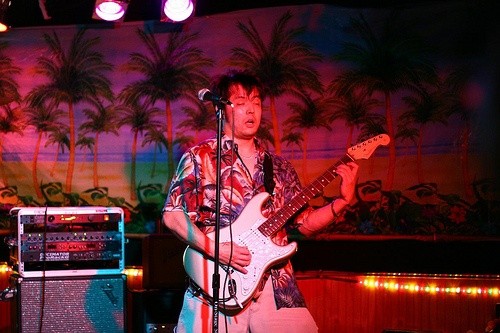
[{"xmin": 198, "ymin": 88, "xmax": 234, "ymax": 108}]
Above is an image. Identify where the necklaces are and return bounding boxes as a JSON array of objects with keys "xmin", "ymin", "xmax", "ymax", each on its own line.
[{"xmin": 239, "ymin": 153, "xmax": 255, "ymax": 159}]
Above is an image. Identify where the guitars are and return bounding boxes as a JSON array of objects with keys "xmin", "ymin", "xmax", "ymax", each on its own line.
[{"xmin": 182, "ymin": 132, "xmax": 392, "ymax": 309}]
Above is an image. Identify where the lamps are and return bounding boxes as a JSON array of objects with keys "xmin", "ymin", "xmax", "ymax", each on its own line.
[
  {"xmin": 92, "ymin": 0, "xmax": 131, "ymax": 24},
  {"xmin": 160, "ymin": 0, "xmax": 197, "ymax": 23}
]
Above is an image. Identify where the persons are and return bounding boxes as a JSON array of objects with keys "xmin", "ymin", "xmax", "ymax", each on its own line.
[
  {"xmin": 162, "ymin": 70, "xmax": 358, "ymax": 333},
  {"xmin": 128, "ymin": 217, "xmax": 181, "ymax": 323}
]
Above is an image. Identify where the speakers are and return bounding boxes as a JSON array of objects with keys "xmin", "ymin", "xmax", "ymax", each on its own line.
[{"xmin": 13, "ymin": 275, "xmax": 127, "ymax": 333}]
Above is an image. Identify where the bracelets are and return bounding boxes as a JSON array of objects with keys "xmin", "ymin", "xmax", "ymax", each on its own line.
[{"xmin": 331, "ymin": 199, "xmax": 344, "ymax": 217}]
